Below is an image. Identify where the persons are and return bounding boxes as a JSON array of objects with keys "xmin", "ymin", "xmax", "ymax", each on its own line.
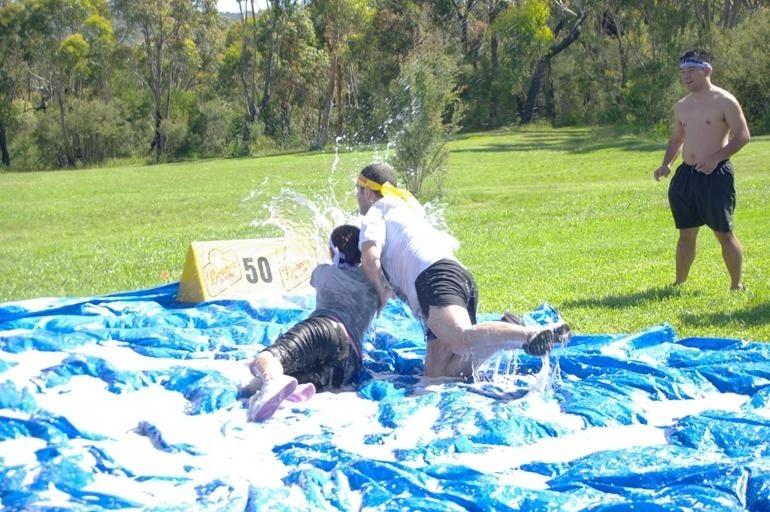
[
  {"xmin": 651, "ymin": 47, "xmax": 753, "ymax": 298},
  {"xmin": 241, "ymin": 224, "xmax": 391, "ymax": 425},
  {"xmin": 353, "ymin": 160, "xmax": 572, "ymax": 387}
]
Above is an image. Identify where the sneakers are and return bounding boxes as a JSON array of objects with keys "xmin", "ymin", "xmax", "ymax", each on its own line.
[
  {"xmin": 501, "ymin": 313, "xmax": 527, "ymax": 325},
  {"xmin": 288, "ymin": 383, "xmax": 315, "ymax": 403},
  {"xmin": 524, "ymin": 324, "xmax": 571, "ymax": 355},
  {"xmin": 252, "ymin": 374, "xmax": 297, "ymax": 421}
]
